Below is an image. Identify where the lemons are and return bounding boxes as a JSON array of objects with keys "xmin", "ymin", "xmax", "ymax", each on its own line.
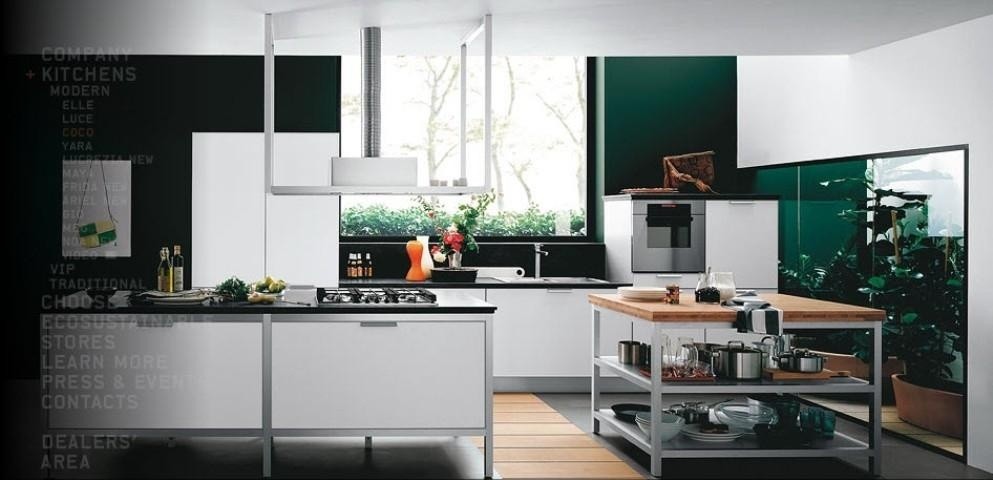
[{"xmin": 255, "ymin": 276, "xmax": 285, "ymax": 293}]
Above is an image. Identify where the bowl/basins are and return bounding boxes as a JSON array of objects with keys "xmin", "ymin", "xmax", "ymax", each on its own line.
[
  {"xmin": 610, "ymin": 402, "xmax": 653, "ymax": 422},
  {"xmin": 635, "ymin": 412, "xmax": 685, "ymax": 443},
  {"xmin": 617, "ymin": 340, "xmax": 647, "ymax": 366}
]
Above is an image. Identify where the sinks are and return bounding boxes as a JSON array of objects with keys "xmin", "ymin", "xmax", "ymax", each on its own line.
[{"xmin": 490, "ymin": 276, "xmax": 611, "ymax": 284}]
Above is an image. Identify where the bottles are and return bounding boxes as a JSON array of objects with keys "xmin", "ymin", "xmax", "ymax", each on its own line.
[
  {"xmin": 660, "ymin": 333, "xmax": 698, "ymax": 379},
  {"xmin": 346, "ymin": 253, "xmax": 374, "ymax": 279},
  {"xmin": 694, "ymin": 273, "xmax": 708, "ymax": 301},
  {"xmin": 157, "ymin": 245, "xmax": 184, "ymax": 293}
]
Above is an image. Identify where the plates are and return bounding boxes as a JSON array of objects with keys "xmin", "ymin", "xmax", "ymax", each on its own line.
[
  {"xmin": 707, "ymin": 401, "xmax": 779, "ymax": 438},
  {"xmin": 616, "ymin": 286, "xmax": 668, "ymax": 301},
  {"xmin": 146, "ymin": 295, "xmax": 206, "ymax": 306},
  {"xmin": 680, "ymin": 424, "xmax": 743, "ymax": 443}
]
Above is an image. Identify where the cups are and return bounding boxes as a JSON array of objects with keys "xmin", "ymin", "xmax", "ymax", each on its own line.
[
  {"xmin": 799, "ymin": 407, "xmax": 835, "ymax": 437},
  {"xmin": 710, "ymin": 271, "xmax": 736, "ymax": 301}
]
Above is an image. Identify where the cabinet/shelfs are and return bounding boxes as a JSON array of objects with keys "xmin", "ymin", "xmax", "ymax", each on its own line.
[
  {"xmin": 485, "ymin": 289, "xmax": 635, "ymax": 379},
  {"xmin": 708, "ymin": 200, "xmax": 780, "ymax": 293},
  {"xmin": 42, "ymin": 321, "xmax": 262, "ymax": 428},
  {"xmin": 606, "ymin": 272, "xmax": 705, "ymax": 331},
  {"xmin": 272, "ymin": 323, "xmax": 486, "ymax": 429}
]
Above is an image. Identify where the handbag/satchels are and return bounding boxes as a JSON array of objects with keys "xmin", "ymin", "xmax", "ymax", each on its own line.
[
  {"xmin": 662, "ymin": 149, "xmax": 720, "ymax": 194},
  {"xmin": 78, "ymin": 219, "xmax": 116, "ymax": 248}
]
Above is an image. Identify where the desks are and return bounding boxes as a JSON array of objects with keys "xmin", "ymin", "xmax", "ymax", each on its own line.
[{"xmin": 584, "ymin": 288, "xmax": 887, "ymax": 480}]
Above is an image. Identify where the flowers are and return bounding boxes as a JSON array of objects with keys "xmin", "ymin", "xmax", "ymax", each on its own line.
[{"xmin": 411, "ymin": 184, "xmax": 505, "ymax": 268}]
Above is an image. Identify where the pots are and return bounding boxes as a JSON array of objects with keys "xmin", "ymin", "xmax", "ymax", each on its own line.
[
  {"xmin": 694, "ymin": 336, "xmax": 825, "ymax": 380},
  {"xmin": 667, "ymin": 400, "xmax": 708, "ymax": 424},
  {"xmin": 749, "ymin": 395, "xmax": 800, "ymax": 436}
]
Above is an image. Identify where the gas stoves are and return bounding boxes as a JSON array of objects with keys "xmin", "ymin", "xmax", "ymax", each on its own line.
[{"xmin": 314, "ymin": 286, "xmax": 437, "ymax": 304}]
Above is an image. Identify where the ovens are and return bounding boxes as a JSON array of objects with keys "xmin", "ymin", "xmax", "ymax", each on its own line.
[{"xmin": 631, "ymin": 196, "xmax": 706, "ymax": 273}]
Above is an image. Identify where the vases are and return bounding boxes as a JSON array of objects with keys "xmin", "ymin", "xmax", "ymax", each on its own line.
[{"xmin": 401, "ymin": 234, "xmax": 462, "ymax": 283}]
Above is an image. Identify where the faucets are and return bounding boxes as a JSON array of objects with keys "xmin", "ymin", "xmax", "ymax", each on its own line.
[{"xmin": 534, "ymin": 243, "xmax": 549, "ymax": 278}]
